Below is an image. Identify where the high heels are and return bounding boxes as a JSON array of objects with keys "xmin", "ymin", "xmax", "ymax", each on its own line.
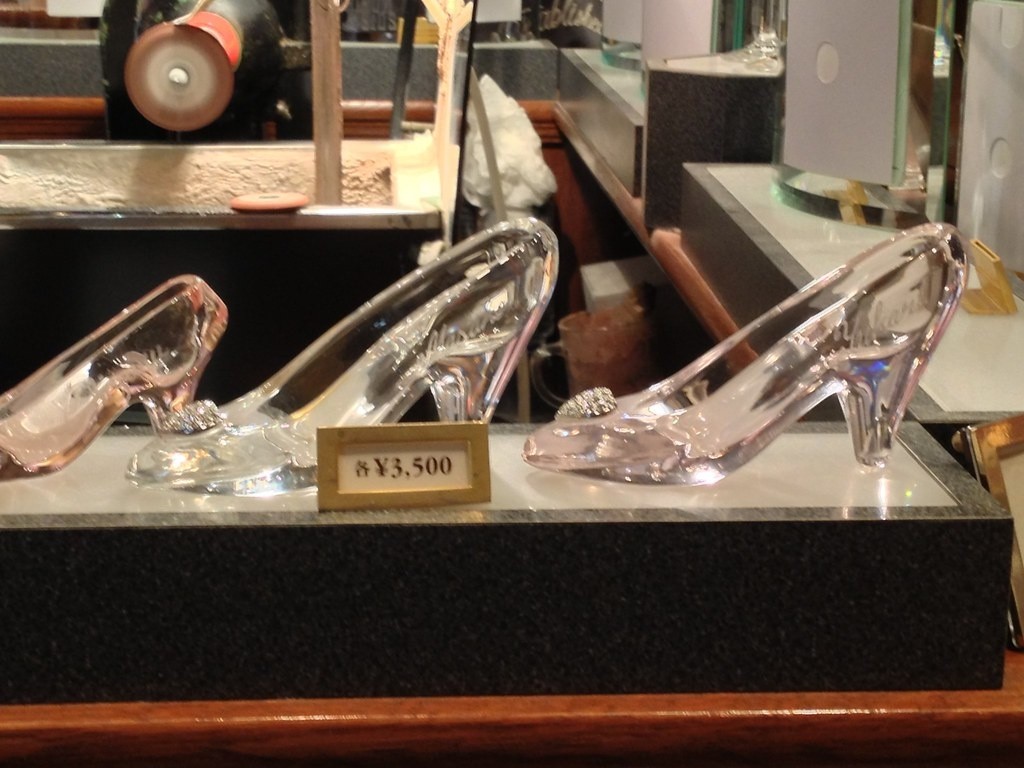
[
  {"xmin": 0, "ymin": 274, "xmax": 229, "ymax": 483},
  {"xmin": 523, "ymin": 221, "xmax": 967, "ymax": 487},
  {"xmin": 125, "ymin": 217, "xmax": 559, "ymax": 498}
]
